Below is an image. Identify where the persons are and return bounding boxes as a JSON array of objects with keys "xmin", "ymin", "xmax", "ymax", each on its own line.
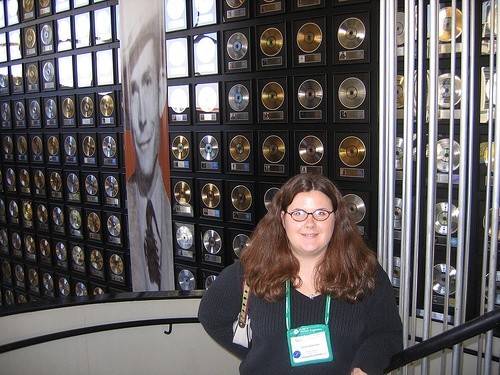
[
  {"xmin": 119, "ymin": 0, "xmax": 175, "ymax": 293},
  {"xmin": 198, "ymin": 174, "xmax": 403, "ymax": 375}
]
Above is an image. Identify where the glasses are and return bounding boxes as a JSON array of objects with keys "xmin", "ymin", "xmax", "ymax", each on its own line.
[{"xmin": 285, "ymin": 209, "xmax": 336, "ymax": 222}]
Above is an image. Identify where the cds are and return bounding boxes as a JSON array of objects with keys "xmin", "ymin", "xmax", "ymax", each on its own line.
[{"xmin": 0, "ymin": 0, "xmax": 497, "ymax": 306}]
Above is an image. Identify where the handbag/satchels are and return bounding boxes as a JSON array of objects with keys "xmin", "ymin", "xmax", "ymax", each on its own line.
[{"xmin": 232, "ymin": 312, "xmax": 252, "ymax": 349}]
